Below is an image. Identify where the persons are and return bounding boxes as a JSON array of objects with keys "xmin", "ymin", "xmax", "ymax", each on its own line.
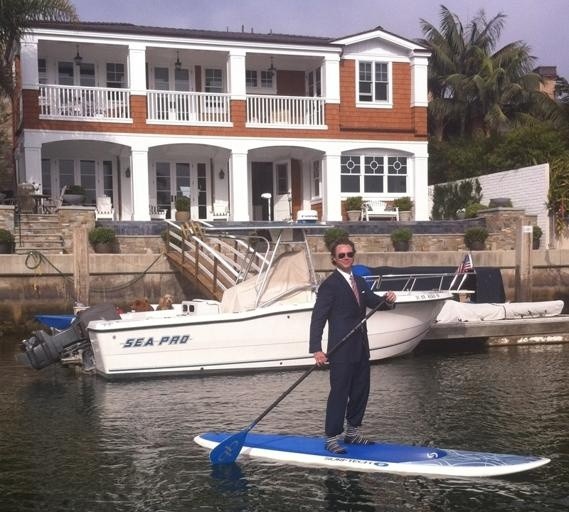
[{"xmin": 310, "ymin": 237, "xmax": 397, "ymax": 454}]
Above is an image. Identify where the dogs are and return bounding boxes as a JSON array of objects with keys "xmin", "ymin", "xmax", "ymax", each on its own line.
[
  {"xmin": 155, "ymin": 294, "xmax": 176, "ymax": 310},
  {"xmin": 127, "ymin": 296, "xmax": 154, "ymax": 311}
]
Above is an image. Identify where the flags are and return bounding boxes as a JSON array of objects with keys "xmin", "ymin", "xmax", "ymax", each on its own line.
[{"xmin": 458, "ymin": 255, "xmax": 470, "ymax": 273}]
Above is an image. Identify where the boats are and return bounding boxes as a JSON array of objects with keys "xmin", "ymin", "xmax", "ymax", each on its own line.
[{"xmin": 18, "ymin": 223, "xmax": 478, "ymax": 388}]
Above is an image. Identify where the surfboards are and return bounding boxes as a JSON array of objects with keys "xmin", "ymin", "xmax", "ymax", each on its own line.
[{"xmin": 193, "ymin": 430, "xmax": 551, "ymax": 478}]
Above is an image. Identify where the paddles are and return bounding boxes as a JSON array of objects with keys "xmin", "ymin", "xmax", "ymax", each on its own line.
[{"xmin": 209, "ymin": 298, "xmax": 387, "ymax": 465}]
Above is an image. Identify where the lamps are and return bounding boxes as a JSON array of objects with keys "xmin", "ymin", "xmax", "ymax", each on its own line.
[
  {"xmin": 126, "ymin": 167, "xmax": 130, "ymax": 178},
  {"xmin": 219, "ymin": 170, "xmax": 226, "ymax": 180},
  {"xmin": 174, "ymin": 51, "xmax": 182, "ymax": 69},
  {"xmin": 267, "ymin": 57, "xmax": 276, "ymax": 77},
  {"xmin": 74, "ymin": 46, "xmax": 82, "ymax": 65}
]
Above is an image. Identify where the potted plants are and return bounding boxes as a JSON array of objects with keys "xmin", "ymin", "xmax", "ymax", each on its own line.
[
  {"xmin": 394, "ymin": 196, "xmax": 414, "ymax": 221},
  {"xmin": 175, "ymin": 196, "xmax": 191, "ymax": 221},
  {"xmin": 89, "ymin": 226, "xmax": 116, "ymax": 253},
  {"xmin": 533, "ymin": 225, "xmax": 544, "ymax": 249},
  {"xmin": 0, "ymin": 230, "xmax": 15, "ymax": 254},
  {"xmin": 347, "ymin": 199, "xmax": 364, "ymax": 221},
  {"xmin": 391, "ymin": 228, "xmax": 414, "ymax": 251},
  {"xmin": 161, "ymin": 229, "xmax": 180, "ymax": 250},
  {"xmin": 465, "ymin": 228, "xmax": 489, "ymax": 250},
  {"xmin": 324, "ymin": 226, "xmax": 348, "ymax": 251},
  {"xmin": 62, "ymin": 184, "xmax": 87, "ymax": 205}
]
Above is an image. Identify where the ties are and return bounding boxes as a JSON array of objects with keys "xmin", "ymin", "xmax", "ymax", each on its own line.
[{"xmin": 349, "ymin": 275, "xmax": 360, "ymax": 306}]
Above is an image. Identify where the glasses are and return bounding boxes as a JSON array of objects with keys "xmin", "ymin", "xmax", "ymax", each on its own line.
[{"xmin": 338, "ymin": 252, "xmax": 354, "ymax": 259}]
